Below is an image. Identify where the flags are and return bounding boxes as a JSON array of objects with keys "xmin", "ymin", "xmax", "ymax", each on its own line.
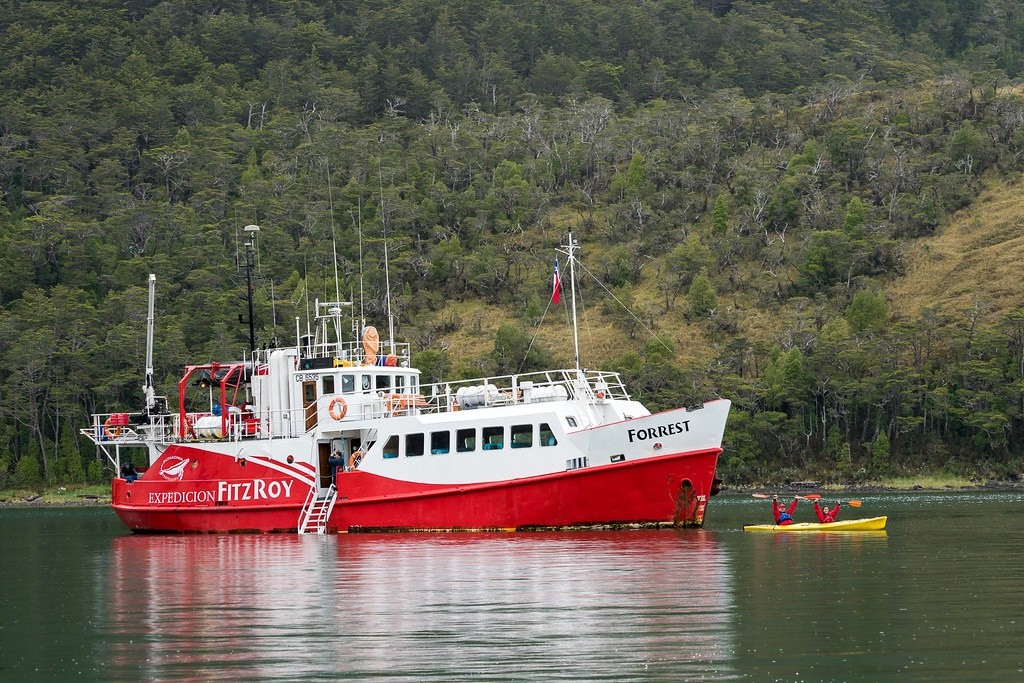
[{"xmin": 552, "ymin": 261, "xmax": 561, "ymax": 305}]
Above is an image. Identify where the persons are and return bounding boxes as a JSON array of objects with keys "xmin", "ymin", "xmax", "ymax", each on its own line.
[
  {"xmin": 773, "ymin": 495, "xmax": 798, "ymax": 525},
  {"xmin": 814, "ymin": 498, "xmax": 840, "ymax": 523},
  {"xmin": 329, "ymin": 448, "xmax": 345, "ymax": 490}
]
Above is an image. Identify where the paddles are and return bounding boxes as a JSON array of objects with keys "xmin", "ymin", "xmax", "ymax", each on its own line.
[
  {"xmin": 795, "ymin": 497, "xmax": 862, "ymax": 507},
  {"xmin": 752, "ymin": 493, "xmax": 821, "ymax": 500}
]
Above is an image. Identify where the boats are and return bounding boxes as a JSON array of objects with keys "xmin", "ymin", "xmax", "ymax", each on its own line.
[
  {"xmin": 79, "ymin": 152, "xmax": 731, "ymax": 537},
  {"xmin": 742, "ymin": 515, "xmax": 888, "ymax": 532}
]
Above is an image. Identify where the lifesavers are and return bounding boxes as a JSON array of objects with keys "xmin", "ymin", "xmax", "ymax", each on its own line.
[
  {"xmin": 104, "ymin": 418, "xmax": 121, "ymax": 439},
  {"xmin": 387, "ymin": 393, "xmax": 405, "ymax": 415},
  {"xmin": 453, "ymin": 400, "xmax": 458, "ymax": 412},
  {"xmin": 597, "ymin": 392, "xmax": 604, "ymax": 398},
  {"xmin": 328, "ymin": 397, "xmax": 347, "ymax": 421},
  {"xmin": 173, "ymin": 420, "xmax": 187, "ymax": 435},
  {"xmin": 349, "ymin": 451, "xmax": 366, "ymax": 471}
]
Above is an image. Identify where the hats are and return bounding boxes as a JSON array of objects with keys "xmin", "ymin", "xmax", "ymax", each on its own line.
[{"xmin": 780, "ymin": 504, "xmax": 785, "ymax": 507}]
